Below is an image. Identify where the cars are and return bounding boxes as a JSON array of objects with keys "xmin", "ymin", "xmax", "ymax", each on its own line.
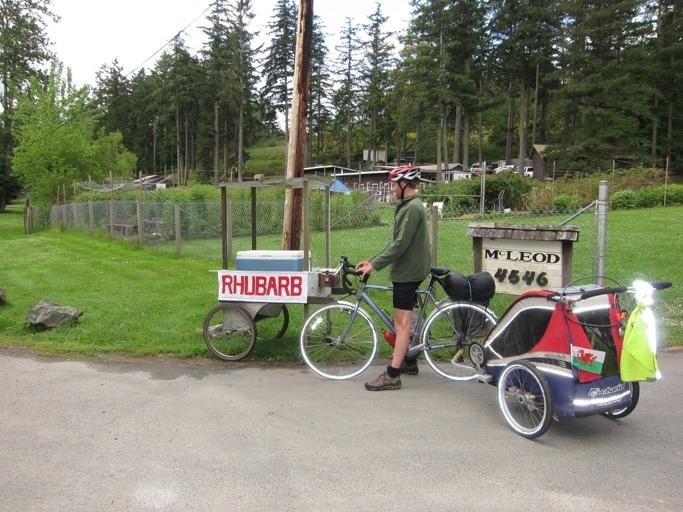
[{"xmin": 473, "ymin": 162, "xmax": 532, "ymax": 178}]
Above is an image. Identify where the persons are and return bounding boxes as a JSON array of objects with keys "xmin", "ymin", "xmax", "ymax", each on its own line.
[{"xmin": 352, "ymin": 163, "xmax": 432, "ymax": 392}]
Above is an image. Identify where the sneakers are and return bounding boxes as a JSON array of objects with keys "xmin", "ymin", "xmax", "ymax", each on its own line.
[
  {"xmin": 365, "ymin": 371, "xmax": 401, "ymax": 390},
  {"xmin": 391, "ymin": 359, "xmax": 418, "ymax": 375}
]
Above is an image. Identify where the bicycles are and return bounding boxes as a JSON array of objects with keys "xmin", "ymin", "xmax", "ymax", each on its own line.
[{"xmin": 300, "ymin": 256, "xmax": 500, "ymax": 380}]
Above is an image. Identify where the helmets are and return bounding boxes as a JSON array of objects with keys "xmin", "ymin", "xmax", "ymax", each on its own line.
[{"xmin": 388, "ymin": 165, "xmax": 423, "ymax": 188}]
